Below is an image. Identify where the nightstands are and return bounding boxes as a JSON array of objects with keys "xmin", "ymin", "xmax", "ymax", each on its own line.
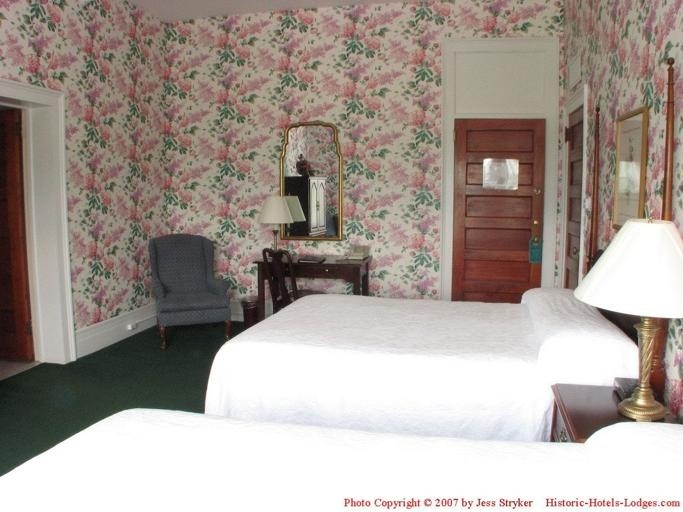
[{"xmin": 550, "ymin": 382, "xmax": 682, "ymax": 441}]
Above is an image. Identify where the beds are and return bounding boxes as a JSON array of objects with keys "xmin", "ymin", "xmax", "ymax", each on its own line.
[
  {"xmin": 204, "ymin": 57, "xmax": 676, "ymax": 440},
  {"xmin": 1, "ymin": 409, "xmax": 681, "ymax": 499}
]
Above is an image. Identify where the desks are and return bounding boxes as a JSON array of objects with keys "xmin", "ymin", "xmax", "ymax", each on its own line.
[{"xmin": 253, "ymin": 255, "xmax": 371, "ymax": 321}]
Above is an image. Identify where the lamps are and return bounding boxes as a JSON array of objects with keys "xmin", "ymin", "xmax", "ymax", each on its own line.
[
  {"xmin": 574, "ymin": 218, "xmax": 683, "ymax": 422},
  {"xmin": 284, "ymin": 195, "xmax": 306, "ymax": 237},
  {"xmin": 259, "ymin": 197, "xmax": 294, "ymax": 249}
]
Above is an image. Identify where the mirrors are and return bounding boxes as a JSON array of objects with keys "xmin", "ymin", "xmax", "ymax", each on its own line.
[{"xmin": 280, "ymin": 121, "xmax": 341, "ymax": 240}]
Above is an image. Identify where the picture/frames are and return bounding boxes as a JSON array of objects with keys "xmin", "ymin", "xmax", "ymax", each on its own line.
[{"xmin": 612, "ymin": 106, "xmax": 649, "ymax": 229}]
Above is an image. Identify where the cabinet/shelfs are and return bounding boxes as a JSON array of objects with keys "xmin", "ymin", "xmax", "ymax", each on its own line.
[{"xmin": 286, "ymin": 175, "xmax": 327, "ymax": 236}]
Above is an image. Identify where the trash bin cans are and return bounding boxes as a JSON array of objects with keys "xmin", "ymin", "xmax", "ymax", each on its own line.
[{"xmin": 242, "ymin": 296, "xmax": 259, "ymax": 328}]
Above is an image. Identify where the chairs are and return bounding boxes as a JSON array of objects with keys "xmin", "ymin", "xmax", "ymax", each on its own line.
[
  {"xmin": 262, "ymin": 249, "xmax": 326, "ymax": 314},
  {"xmin": 148, "ymin": 235, "xmax": 232, "ymax": 349}
]
